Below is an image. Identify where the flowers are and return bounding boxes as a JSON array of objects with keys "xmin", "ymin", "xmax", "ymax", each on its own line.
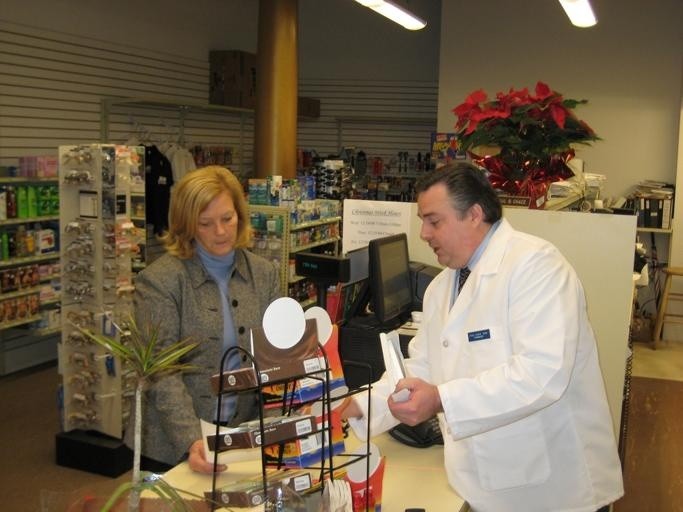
[{"xmin": 448, "ymin": 82, "xmax": 598, "ymax": 209}]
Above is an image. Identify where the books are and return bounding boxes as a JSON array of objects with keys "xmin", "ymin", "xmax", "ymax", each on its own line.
[{"xmin": 632, "ymin": 179, "xmax": 675, "ymax": 200}]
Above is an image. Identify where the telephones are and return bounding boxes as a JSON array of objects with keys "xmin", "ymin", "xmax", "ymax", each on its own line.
[{"xmin": 388, "ymin": 415, "xmax": 445, "ymax": 448}]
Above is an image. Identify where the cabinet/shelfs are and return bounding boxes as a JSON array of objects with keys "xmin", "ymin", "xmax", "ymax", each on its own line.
[
  {"xmin": 54, "ymin": 144, "xmax": 233, "ymax": 480},
  {"xmin": 1, "ymin": 141, "xmax": 61, "ymax": 377},
  {"xmin": 233, "ymin": 144, "xmax": 434, "ymax": 311}
]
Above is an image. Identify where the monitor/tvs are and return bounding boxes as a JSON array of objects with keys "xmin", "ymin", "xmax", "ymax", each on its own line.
[{"xmin": 344, "ymin": 233, "xmax": 415, "ymax": 324}]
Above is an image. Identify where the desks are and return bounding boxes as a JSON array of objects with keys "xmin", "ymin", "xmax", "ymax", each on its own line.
[
  {"xmin": 638, "ymin": 225, "xmax": 671, "ymax": 330},
  {"xmin": 129, "ymin": 322, "xmax": 470, "ymax": 510}
]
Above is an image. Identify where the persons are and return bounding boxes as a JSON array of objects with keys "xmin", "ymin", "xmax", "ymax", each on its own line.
[
  {"xmin": 327, "ymin": 160, "xmax": 627, "ymax": 510},
  {"xmin": 112, "ymin": 164, "xmax": 286, "ymax": 477}
]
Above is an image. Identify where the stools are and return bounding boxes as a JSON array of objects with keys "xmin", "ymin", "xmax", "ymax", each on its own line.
[{"xmin": 653, "ymin": 266, "xmax": 683, "ymax": 351}]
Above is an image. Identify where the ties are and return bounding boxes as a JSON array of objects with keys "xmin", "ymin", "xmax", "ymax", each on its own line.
[{"xmin": 458, "ymin": 266, "xmax": 471, "ymax": 295}]
